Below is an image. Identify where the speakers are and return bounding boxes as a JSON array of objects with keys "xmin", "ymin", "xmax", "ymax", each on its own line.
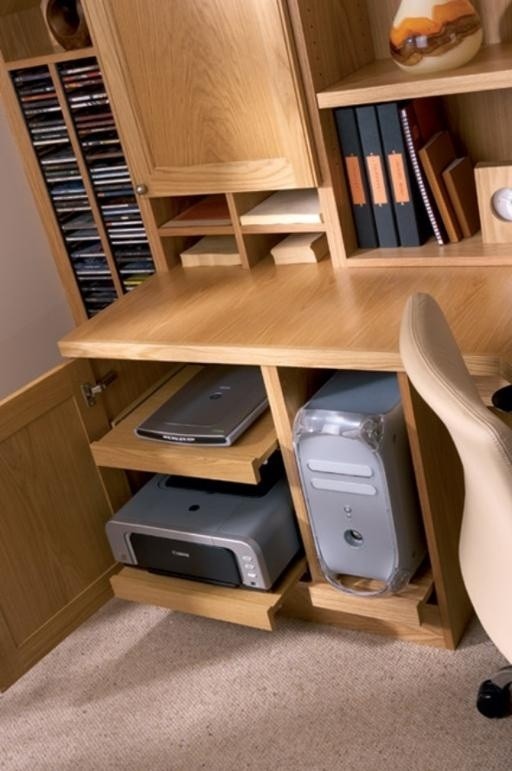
[{"xmin": 38, "ymin": 0, "xmax": 94, "ymax": 53}]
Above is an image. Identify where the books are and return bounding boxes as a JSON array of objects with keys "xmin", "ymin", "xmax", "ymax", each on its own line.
[
  {"xmin": 17, "ymin": 64, "xmax": 155, "ymax": 318},
  {"xmin": 336, "ymin": 101, "xmax": 479, "ymax": 248}
]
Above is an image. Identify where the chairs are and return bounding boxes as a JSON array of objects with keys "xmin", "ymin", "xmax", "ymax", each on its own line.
[{"xmin": 398, "ymin": 292, "xmax": 512, "ymax": 717}]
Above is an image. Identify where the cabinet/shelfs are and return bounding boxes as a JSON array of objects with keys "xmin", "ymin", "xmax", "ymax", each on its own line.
[{"xmin": 0, "ymin": 0, "xmax": 512, "ymax": 651}]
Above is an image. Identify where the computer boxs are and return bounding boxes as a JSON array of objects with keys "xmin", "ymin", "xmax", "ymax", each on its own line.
[{"xmin": 290, "ymin": 371, "xmax": 429, "ymax": 599}]
